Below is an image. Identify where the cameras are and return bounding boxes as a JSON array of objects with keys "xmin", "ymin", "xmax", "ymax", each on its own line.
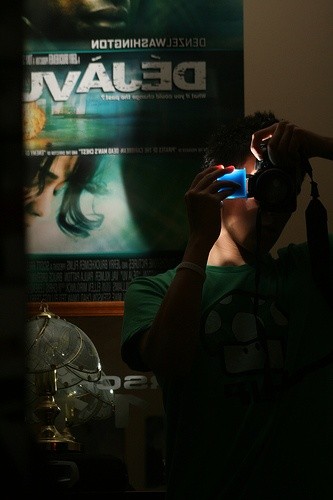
[{"xmin": 214, "ymin": 147, "xmax": 309, "ymax": 213}]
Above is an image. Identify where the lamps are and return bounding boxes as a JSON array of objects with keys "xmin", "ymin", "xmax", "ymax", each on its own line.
[{"xmin": 6, "ymin": 304, "xmax": 119, "ymax": 486}]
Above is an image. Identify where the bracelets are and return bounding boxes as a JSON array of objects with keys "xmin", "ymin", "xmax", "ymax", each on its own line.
[{"xmin": 175, "ymin": 263, "xmax": 208, "ymax": 283}]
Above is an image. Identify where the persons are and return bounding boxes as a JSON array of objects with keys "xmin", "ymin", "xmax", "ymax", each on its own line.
[
  {"xmin": 49, "ymin": 0, "xmax": 134, "ymax": 46},
  {"xmin": 120, "ymin": 109, "xmax": 333, "ymax": 500},
  {"xmin": 25, "ymin": 153, "xmax": 108, "ymax": 239}
]
